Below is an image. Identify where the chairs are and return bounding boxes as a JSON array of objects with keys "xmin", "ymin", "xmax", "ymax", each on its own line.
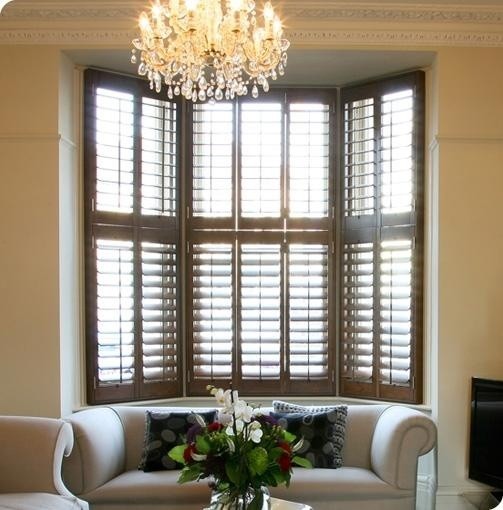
[{"xmin": 0, "ymin": 414, "xmax": 90, "ymax": 510}]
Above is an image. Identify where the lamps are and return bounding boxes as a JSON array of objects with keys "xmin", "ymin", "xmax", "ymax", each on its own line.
[{"xmin": 130, "ymin": 0, "xmax": 290, "ymax": 102}]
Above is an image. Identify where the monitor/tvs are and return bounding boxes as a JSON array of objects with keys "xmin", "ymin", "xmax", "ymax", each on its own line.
[{"xmin": 468, "ymin": 376, "xmax": 503, "ymax": 503}]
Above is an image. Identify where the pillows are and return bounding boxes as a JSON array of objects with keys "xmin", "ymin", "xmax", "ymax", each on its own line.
[{"xmin": 142, "ymin": 400, "xmax": 347, "ymax": 472}]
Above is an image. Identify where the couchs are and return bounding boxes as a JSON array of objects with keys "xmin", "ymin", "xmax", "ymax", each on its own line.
[{"xmin": 61, "ymin": 400, "xmax": 438, "ymax": 510}]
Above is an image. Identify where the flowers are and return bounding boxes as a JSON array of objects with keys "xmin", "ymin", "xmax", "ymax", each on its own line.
[{"xmin": 167, "ymin": 384, "xmax": 313, "ymax": 510}]
